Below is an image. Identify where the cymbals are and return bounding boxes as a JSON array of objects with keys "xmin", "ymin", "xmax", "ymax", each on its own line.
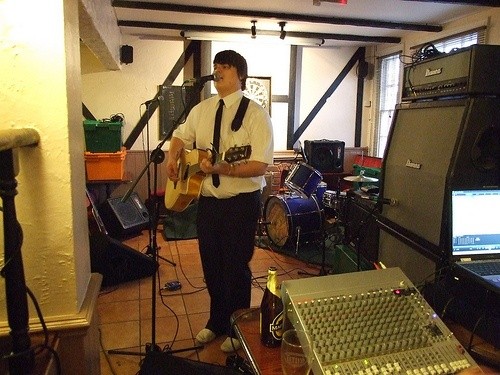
[{"xmin": 343, "ymin": 175, "xmax": 379, "ymax": 182}]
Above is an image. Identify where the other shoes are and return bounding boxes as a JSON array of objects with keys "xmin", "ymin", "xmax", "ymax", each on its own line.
[
  {"xmin": 195, "ymin": 327, "xmax": 217, "ymax": 344},
  {"xmin": 220, "ymin": 336, "xmax": 241, "ymax": 352}
]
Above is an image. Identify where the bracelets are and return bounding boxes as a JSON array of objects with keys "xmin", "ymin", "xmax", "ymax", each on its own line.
[{"xmin": 227, "ymin": 164, "xmax": 233, "ymax": 175}]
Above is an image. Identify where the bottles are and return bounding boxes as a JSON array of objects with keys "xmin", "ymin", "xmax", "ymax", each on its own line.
[{"xmin": 260, "ymin": 266, "xmax": 284, "ymax": 347}]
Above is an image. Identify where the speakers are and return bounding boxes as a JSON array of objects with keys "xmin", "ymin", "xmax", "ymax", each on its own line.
[
  {"xmin": 304, "ymin": 140, "xmax": 345, "ymax": 174},
  {"xmin": 358, "ymin": 61, "xmax": 368, "ymax": 77},
  {"xmin": 98, "ymin": 192, "xmax": 151, "ymax": 241},
  {"xmin": 341, "ymin": 191, "xmax": 383, "ymax": 262},
  {"xmin": 121, "ymin": 45, "xmax": 133, "ymax": 63},
  {"xmin": 90, "ymin": 232, "xmax": 159, "ymax": 287},
  {"xmin": 375, "ymin": 219, "xmax": 447, "ymax": 305},
  {"xmin": 377, "ymin": 94, "xmax": 500, "ymax": 255}
]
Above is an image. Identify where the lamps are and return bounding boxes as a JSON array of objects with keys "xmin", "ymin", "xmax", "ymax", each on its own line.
[
  {"xmin": 250, "ymin": 20, "xmax": 258, "ymax": 38},
  {"xmin": 278, "ymin": 21, "xmax": 288, "ymax": 39}
]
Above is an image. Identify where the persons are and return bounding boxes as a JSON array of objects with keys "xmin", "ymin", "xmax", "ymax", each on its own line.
[{"xmin": 166, "ymin": 49, "xmax": 274, "ymax": 351}]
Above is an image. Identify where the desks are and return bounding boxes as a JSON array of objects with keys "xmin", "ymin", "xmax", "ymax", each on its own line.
[
  {"xmin": 230, "ymin": 301, "xmax": 500, "ymax": 375},
  {"xmin": 86, "ymin": 179, "xmax": 131, "ymax": 202}
]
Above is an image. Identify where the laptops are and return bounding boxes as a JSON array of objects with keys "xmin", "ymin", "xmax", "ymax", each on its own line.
[{"xmin": 449, "ymin": 188, "xmax": 500, "ymax": 291}]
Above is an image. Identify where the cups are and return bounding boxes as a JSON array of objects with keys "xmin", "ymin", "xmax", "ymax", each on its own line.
[{"xmin": 281, "ymin": 330, "xmax": 311, "ymax": 375}]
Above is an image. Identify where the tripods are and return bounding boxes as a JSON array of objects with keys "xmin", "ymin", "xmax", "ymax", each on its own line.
[{"xmin": 108, "ymin": 83, "xmax": 206, "ymax": 362}]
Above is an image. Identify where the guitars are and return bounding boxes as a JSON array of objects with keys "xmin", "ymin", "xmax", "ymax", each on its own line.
[{"xmin": 164, "ymin": 142, "xmax": 253, "ymax": 212}]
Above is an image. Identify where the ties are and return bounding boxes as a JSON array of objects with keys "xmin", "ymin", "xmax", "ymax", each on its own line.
[{"xmin": 212, "ymin": 99, "xmax": 226, "ymax": 188}]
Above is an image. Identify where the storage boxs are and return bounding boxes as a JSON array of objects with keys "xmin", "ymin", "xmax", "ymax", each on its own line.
[
  {"xmin": 84, "ymin": 147, "xmax": 129, "ymax": 181},
  {"xmin": 83, "ymin": 119, "xmax": 124, "ymax": 152}
]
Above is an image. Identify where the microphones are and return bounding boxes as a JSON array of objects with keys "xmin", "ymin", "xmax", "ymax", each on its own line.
[
  {"xmin": 378, "ymin": 197, "xmax": 399, "ymax": 207},
  {"xmin": 185, "ymin": 73, "xmax": 221, "ymax": 83},
  {"xmin": 141, "ymin": 96, "xmax": 164, "ymax": 105},
  {"xmin": 258, "ymin": 221, "xmax": 276, "ymax": 224}
]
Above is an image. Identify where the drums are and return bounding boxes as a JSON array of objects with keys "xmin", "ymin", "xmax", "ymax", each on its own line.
[
  {"xmin": 316, "ymin": 182, "xmax": 327, "ymax": 200},
  {"xmin": 263, "ymin": 192, "xmax": 325, "ymax": 250},
  {"xmin": 285, "ymin": 161, "xmax": 323, "ymax": 200},
  {"xmin": 324, "ymin": 191, "xmax": 347, "ymax": 209}
]
